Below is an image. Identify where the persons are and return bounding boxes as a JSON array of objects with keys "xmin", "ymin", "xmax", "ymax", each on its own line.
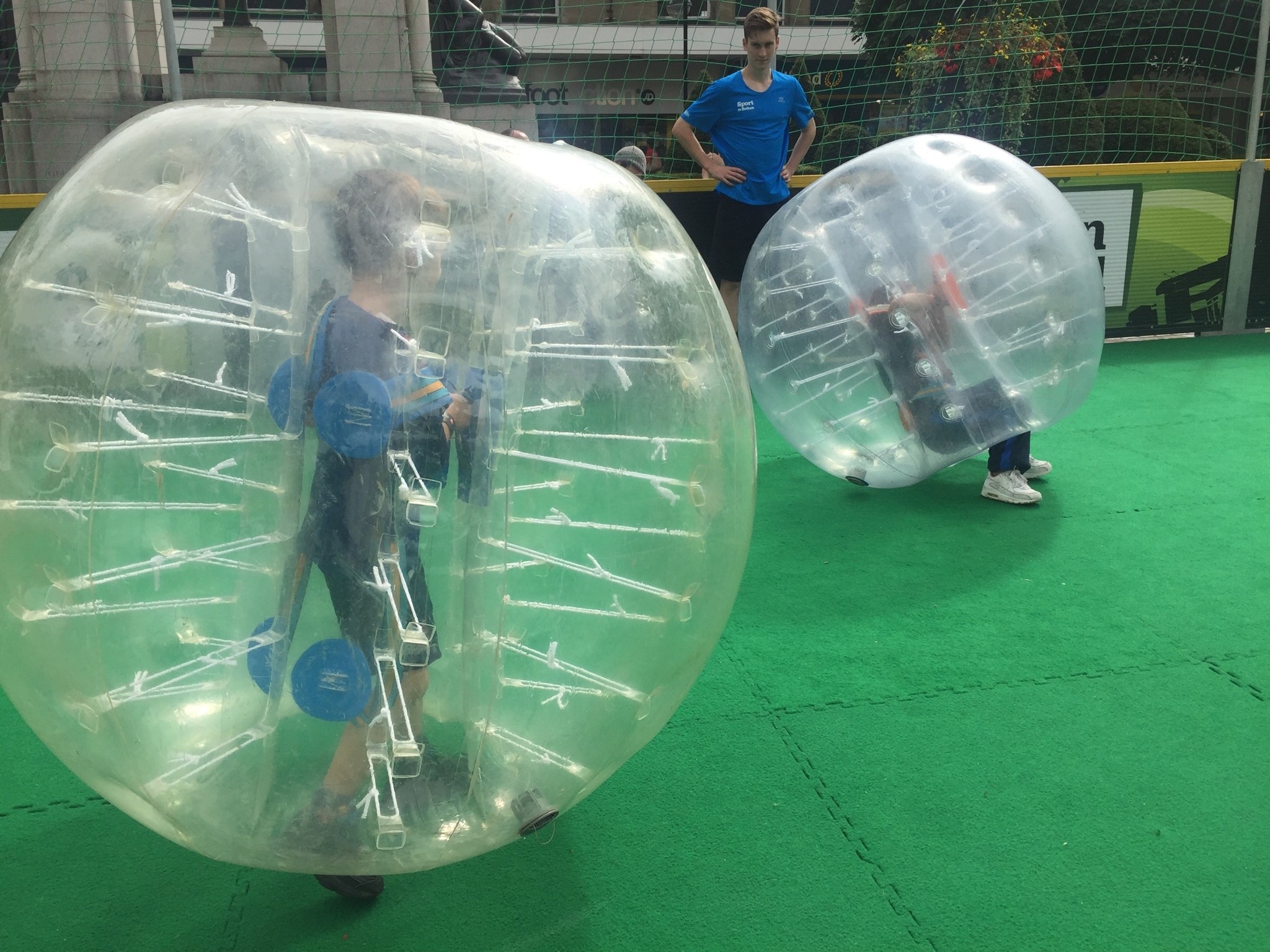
[
  {"xmin": 611, "ymin": 145, "xmax": 648, "ymax": 186},
  {"xmin": 500, "ymin": 127, "xmax": 530, "ymax": 141},
  {"xmin": 672, "ymin": 5, "xmax": 818, "ymax": 341},
  {"xmin": 864, "ymin": 285, "xmax": 1060, "ymax": 509},
  {"xmin": 257, "ymin": 169, "xmax": 501, "ymax": 901},
  {"xmin": 605, "ymin": 135, "xmax": 664, "ymax": 176}
]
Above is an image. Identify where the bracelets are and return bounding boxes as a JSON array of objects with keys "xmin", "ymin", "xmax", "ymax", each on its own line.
[{"xmin": 442, "ymin": 410, "xmax": 457, "ymax": 439}]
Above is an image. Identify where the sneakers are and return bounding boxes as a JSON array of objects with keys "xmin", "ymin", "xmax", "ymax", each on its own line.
[
  {"xmin": 387, "ymin": 758, "xmax": 475, "ymax": 812},
  {"xmin": 1022, "ymin": 456, "xmax": 1052, "ymax": 479},
  {"xmin": 277, "ymin": 791, "xmax": 384, "ymax": 901},
  {"xmin": 981, "ymin": 466, "xmax": 1042, "ymax": 504}
]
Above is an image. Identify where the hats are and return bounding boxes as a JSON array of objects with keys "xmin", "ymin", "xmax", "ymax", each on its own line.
[{"xmin": 613, "ymin": 146, "xmax": 647, "ymax": 180}]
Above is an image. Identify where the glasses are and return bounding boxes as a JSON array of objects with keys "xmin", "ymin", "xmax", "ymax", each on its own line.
[{"xmin": 620, "ymin": 163, "xmax": 628, "ymax": 168}]
